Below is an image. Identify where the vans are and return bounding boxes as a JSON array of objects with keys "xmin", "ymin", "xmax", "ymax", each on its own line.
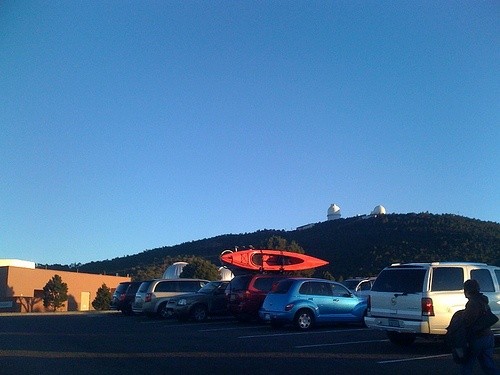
[
  {"xmin": 131, "ymin": 278, "xmax": 214, "ymax": 319},
  {"xmin": 363, "ymin": 261, "xmax": 500, "ymax": 345},
  {"xmin": 109, "ymin": 282, "xmax": 144, "ymax": 315}
]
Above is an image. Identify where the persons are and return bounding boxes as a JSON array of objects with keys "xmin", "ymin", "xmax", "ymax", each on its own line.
[{"xmin": 459, "ymin": 279, "xmax": 500, "ymax": 375}]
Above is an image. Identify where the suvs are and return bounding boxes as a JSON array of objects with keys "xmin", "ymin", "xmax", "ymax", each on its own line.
[{"xmin": 219, "ymin": 274, "xmax": 307, "ymax": 324}]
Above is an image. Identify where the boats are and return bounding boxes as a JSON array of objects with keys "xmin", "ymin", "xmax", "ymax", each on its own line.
[{"xmin": 220, "ymin": 250, "xmax": 330, "ymax": 271}]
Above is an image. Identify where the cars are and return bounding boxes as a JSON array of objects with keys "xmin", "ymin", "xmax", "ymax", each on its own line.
[
  {"xmin": 258, "ymin": 278, "xmax": 370, "ymax": 331},
  {"xmin": 172, "ymin": 281, "xmax": 232, "ymax": 323},
  {"xmin": 340, "ymin": 277, "xmax": 377, "ymax": 300}
]
